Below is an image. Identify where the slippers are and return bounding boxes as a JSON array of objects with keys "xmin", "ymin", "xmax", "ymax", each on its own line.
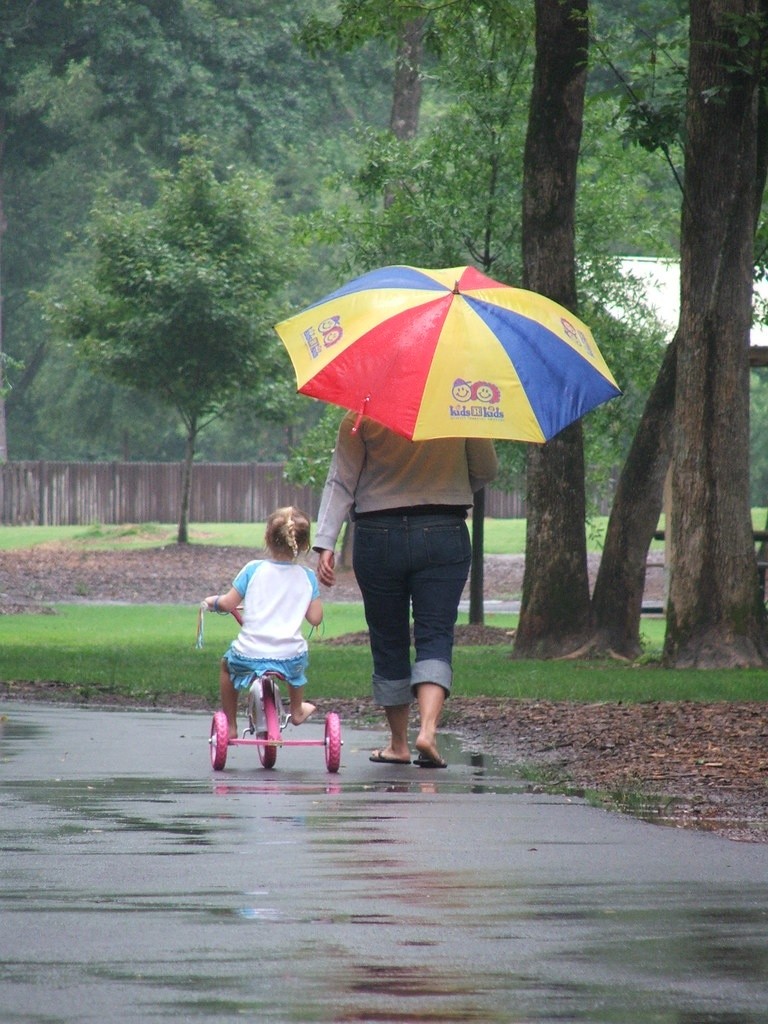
[
  {"xmin": 369, "ymin": 751, "xmax": 411, "ymax": 764},
  {"xmin": 413, "ymin": 753, "xmax": 447, "ymax": 768}
]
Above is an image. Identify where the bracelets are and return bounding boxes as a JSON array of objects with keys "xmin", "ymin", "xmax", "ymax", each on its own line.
[{"xmin": 215, "ymin": 595, "xmax": 229, "ymax": 616}]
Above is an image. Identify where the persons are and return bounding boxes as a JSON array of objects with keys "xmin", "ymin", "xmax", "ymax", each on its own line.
[
  {"xmin": 206, "ymin": 506, "xmax": 322, "ymax": 739},
  {"xmin": 312, "ymin": 407, "xmax": 497, "ymax": 764}
]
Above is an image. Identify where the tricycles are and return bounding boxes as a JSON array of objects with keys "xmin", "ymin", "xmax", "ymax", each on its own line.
[{"xmin": 198, "ymin": 596, "xmax": 344, "ymax": 772}]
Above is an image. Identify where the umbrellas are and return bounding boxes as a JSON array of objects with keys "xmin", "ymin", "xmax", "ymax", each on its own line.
[{"xmin": 273, "ymin": 265, "xmax": 624, "ymax": 444}]
